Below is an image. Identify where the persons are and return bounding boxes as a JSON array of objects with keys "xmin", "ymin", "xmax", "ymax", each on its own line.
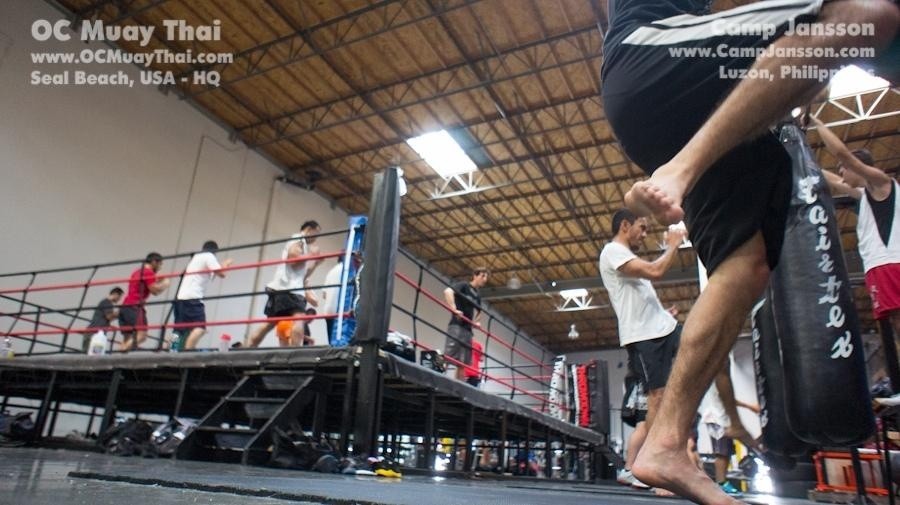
[
  {"xmin": 303, "ymin": 307, "xmax": 318, "ymax": 346},
  {"xmin": 598, "ymin": 209, "xmax": 760, "ymax": 499},
  {"xmin": 242, "ymin": 219, "xmax": 326, "ymax": 348},
  {"xmin": 276, "ymin": 314, "xmax": 295, "ymax": 348},
  {"xmin": 322, "ymin": 253, "xmax": 345, "ymax": 345},
  {"xmin": 116, "ymin": 251, "xmax": 172, "ymax": 353},
  {"xmin": 80, "ymin": 285, "xmax": 124, "ymax": 356},
  {"xmin": 157, "ymin": 239, "xmax": 233, "ymax": 353},
  {"xmin": 615, "ymin": 303, "xmax": 680, "ymax": 491},
  {"xmin": 701, "ymin": 378, "xmax": 761, "ymax": 498},
  {"xmin": 463, "ymin": 331, "xmax": 484, "ymax": 388},
  {"xmin": 597, "ymin": 0, "xmax": 900, "ymax": 505},
  {"xmin": 799, "ymin": 110, "xmax": 900, "ymax": 408},
  {"xmin": 441, "ymin": 267, "xmax": 490, "ymax": 382}
]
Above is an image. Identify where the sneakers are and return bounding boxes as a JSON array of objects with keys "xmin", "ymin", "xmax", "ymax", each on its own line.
[
  {"xmin": 617, "ymin": 469, "xmax": 651, "ymax": 489},
  {"xmin": 340, "ymin": 455, "xmax": 402, "ymax": 477},
  {"xmin": 717, "ymin": 481, "xmax": 743, "ymax": 497}
]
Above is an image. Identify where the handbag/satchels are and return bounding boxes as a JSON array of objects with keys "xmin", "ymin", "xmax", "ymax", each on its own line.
[
  {"xmin": 100, "ymin": 419, "xmax": 151, "ymax": 456},
  {"xmin": 149, "ymin": 417, "xmax": 196, "ymax": 455},
  {"xmin": 0, "ymin": 408, "xmax": 34, "ymax": 441},
  {"xmin": 267, "ymin": 424, "xmax": 340, "ymax": 473}
]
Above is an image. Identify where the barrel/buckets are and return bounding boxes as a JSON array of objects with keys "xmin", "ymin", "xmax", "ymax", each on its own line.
[{"xmin": 0, "ymin": 338, "xmax": 15, "ymax": 360}]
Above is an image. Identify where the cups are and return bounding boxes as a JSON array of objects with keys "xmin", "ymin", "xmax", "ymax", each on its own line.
[{"xmin": 219, "ymin": 334, "xmax": 231, "ymax": 352}]
